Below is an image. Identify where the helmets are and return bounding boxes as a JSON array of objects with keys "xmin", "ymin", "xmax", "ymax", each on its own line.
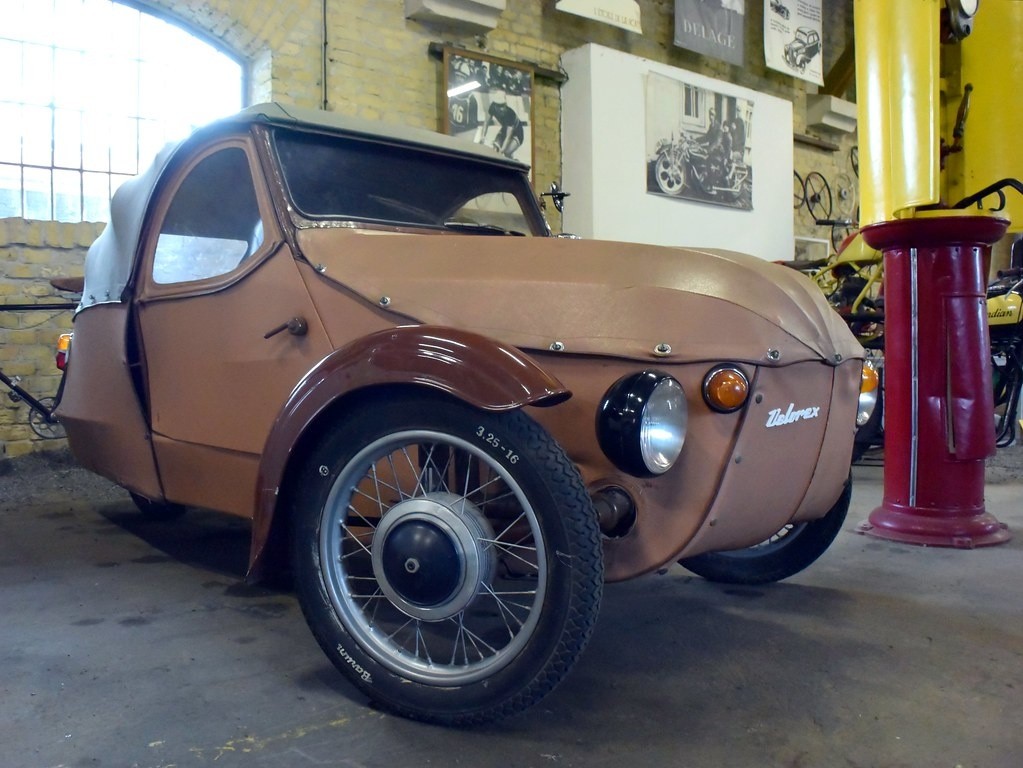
[{"xmin": 493, "ymin": 91, "xmax": 506, "ymax": 103}]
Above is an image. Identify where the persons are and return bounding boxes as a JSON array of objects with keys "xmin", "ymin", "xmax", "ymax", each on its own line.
[
  {"xmin": 696, "ymin": 107, "xmax": 742, "ymax": 202},
  {"xmin": 448, "ymin": 56, "xmax": 525, "ymax": 160}
]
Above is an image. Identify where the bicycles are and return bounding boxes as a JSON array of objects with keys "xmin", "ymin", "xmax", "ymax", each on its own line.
[{"xmin": 0, "ymin": 276, "xmax": 83, "ymax": 439}]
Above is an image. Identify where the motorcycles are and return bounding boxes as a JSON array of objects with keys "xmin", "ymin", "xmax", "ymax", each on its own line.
[
  {"xmin": 654, "ymin": 128, "xmax": 748, "ymax": 202},
  {"xmin": 763, "ymin": 178, "xmax": 1023, "ymax": 462}
]
